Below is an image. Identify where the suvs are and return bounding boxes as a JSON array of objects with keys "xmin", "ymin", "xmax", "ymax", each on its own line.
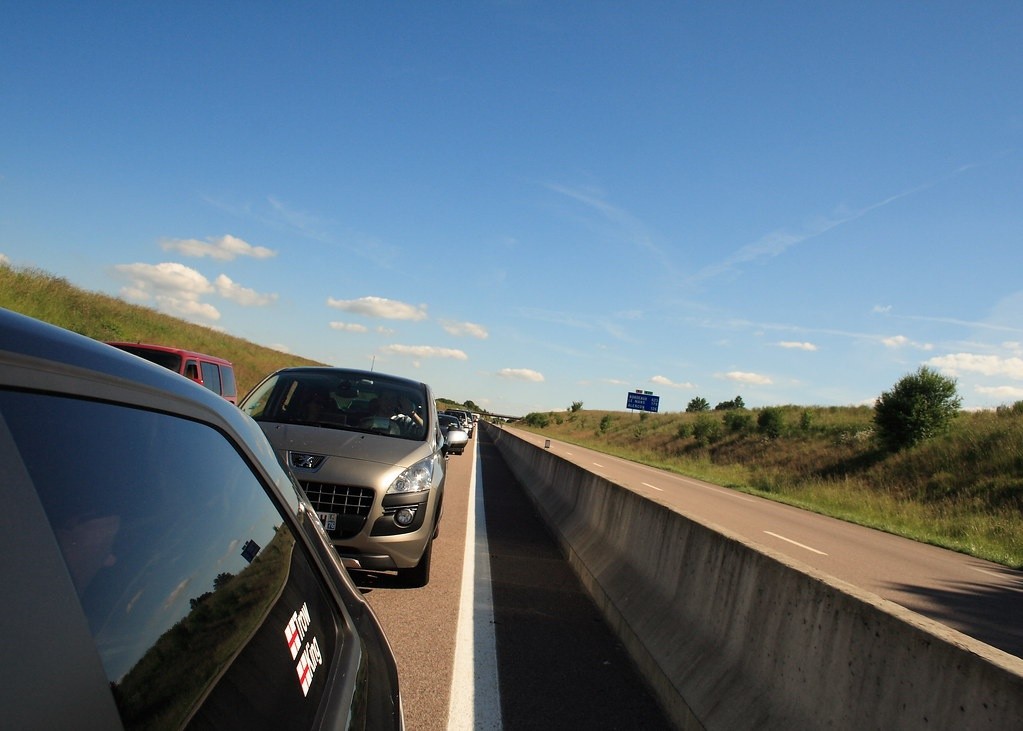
[
  {"xmin": 233, "ymin": 365, "xmax": 470, "ymax": 587},
  {"xmin": 100, "ymin": 340, "xmax": 237, "ymax": 407}
]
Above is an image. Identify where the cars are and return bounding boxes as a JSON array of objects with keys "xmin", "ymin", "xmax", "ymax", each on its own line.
[
  {"xmin": 444, "ymin": 408, "xmax": 477, "ymax": 439},
  {"xmin": 0, "ymin": 308, "xmax": 408, "ymax": 731},
  {"xmin": 437, "ymin": 413, "xmax": 463, "ymax": 456}
]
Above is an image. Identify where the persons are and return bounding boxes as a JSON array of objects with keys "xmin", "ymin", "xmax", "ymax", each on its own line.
[{"xmin": 288, "ymin": 387, "xmax": 424, "ymax": 435}]
